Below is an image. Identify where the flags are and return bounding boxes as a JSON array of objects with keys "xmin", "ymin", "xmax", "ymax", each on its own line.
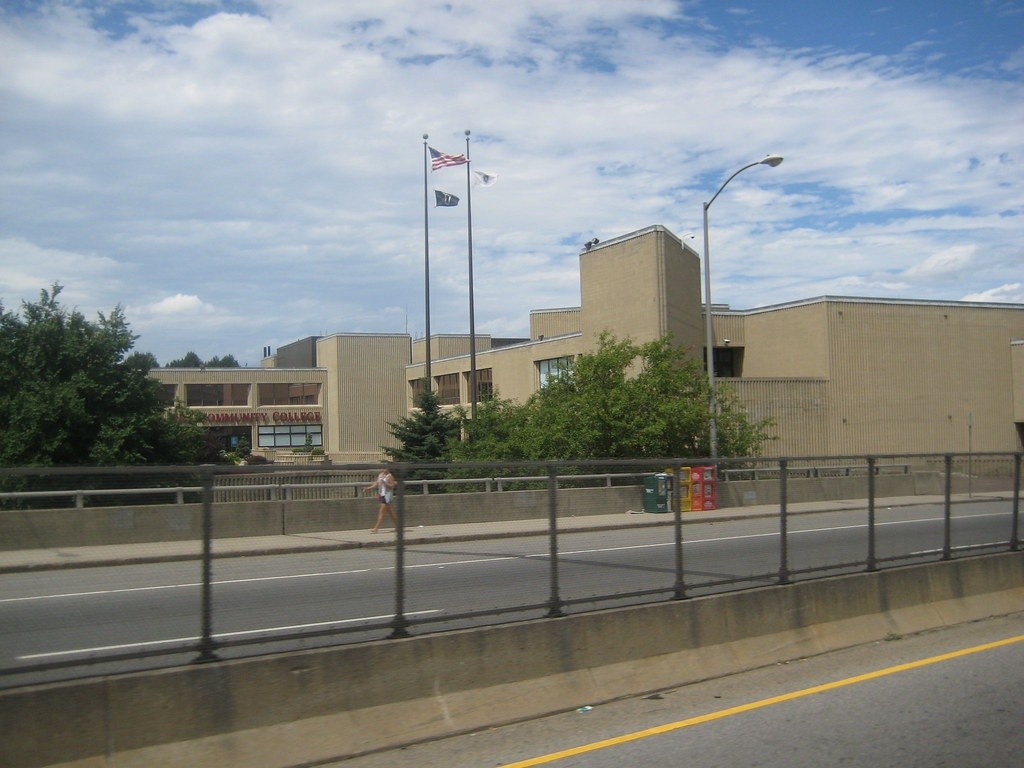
[
  {"xmin": 435, "ymin": 190, "xmax": 461, "ymax": 207},
  {"xmin": 429, "ymin": 147, "xmax": 471, "ymax": 172},
  {"xmin": 469, "ymin": 170, "xmax": 500, "ymax": 188}
]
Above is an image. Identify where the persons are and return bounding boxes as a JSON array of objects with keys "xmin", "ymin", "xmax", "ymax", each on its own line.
[{"xmin": 361, "ymin": 460, "xmax": 397, "ymax": 533}]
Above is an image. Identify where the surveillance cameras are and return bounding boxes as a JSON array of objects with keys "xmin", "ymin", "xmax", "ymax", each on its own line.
[
  {"xmin": 690, "ymin": 235, "xmax": 695, "ymax": 238},
  {"xmin": 724, "ymin": 339, "xmax": 731, "ymax": 344}
]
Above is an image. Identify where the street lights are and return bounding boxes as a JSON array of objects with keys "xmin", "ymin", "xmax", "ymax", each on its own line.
[{"xmin": 701, "ymin": 153, "xmax": 783, "ymax": 478}]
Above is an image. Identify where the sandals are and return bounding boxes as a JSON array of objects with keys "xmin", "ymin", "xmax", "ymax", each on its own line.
[
  {"xmin": 391, "ymin": 528, "xmax": 397, "ymax": 532},
  {"xmin": 370, "ymin": 528, "xmax": 377, "ymax": 534}
]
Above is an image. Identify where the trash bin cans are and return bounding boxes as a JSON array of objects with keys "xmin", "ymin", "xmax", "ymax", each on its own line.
[{"xmin": 643, "ymin": 465, "xmax": 717, "ymax": 513}]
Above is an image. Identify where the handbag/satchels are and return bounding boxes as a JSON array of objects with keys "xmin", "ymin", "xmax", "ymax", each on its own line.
[{"xmin": 384, "ymin": 492, "xmax": 394, "ymax": 503}]
